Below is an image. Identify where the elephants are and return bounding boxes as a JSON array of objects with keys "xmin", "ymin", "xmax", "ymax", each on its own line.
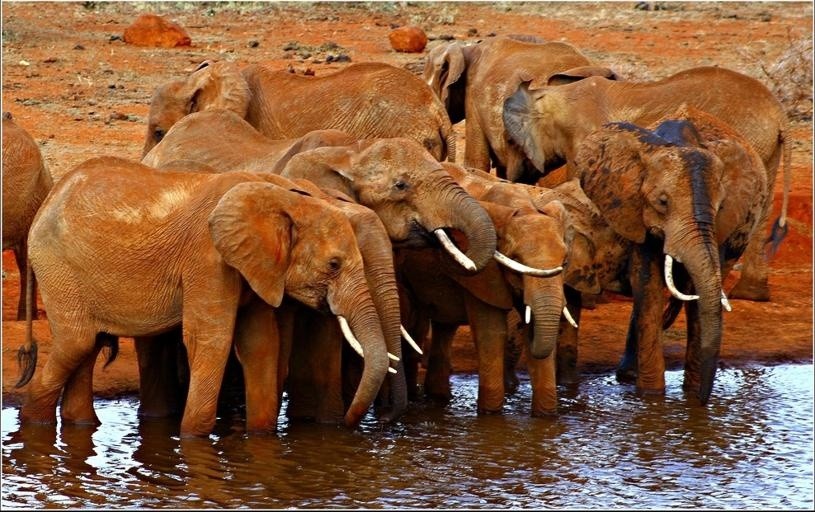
[{"xmin": 2, "ymin": 36, "xmax": 792, "ymax": 438}]
